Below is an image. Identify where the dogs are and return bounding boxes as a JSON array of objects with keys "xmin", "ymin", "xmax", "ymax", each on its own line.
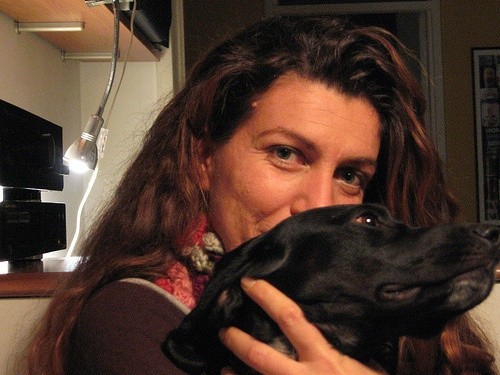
[{"xmin": 159, "ymin": 202, "xmax": 495, "ymax": 374}]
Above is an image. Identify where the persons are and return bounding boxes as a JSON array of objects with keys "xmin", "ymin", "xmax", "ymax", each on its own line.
[{"xmin": 20, "ymin": 8, "xmax": 500, "ymax": 374}]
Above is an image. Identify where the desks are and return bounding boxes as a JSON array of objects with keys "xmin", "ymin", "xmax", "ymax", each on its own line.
[{"xmin": 0, "ymin": 260, "xmax": 90, "ymax": 373}]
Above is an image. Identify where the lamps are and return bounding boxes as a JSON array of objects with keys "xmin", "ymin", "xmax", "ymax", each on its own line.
[{"xmin": 62, "ymin": 0, "xmax": 136, "ymax": 175}]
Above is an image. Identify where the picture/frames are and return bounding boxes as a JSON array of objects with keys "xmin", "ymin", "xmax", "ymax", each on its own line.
[{"xmin": 468, "ymin": 45, "xmax": 500, "ymax": 224}]
